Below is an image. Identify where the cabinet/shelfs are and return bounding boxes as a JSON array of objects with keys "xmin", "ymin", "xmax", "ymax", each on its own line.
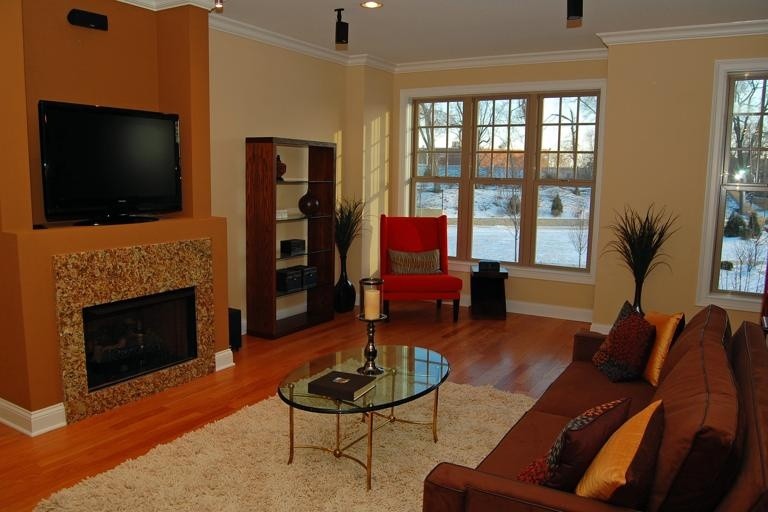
[{"xmin": 245, "ymin": 136, "xmax": 338, "ymax": 340}]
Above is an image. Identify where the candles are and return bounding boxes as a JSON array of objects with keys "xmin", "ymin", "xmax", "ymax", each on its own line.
[{"xmin": 364, "ymin": 290, "xmax": 380, "ymax": 320}]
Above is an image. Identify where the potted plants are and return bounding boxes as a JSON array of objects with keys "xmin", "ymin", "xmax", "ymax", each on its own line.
[
  {"xmin": 600, "ymin": 203, "xmax": 683, "ymax": 314},
  {"xmin": 335, "ymin": 195, "xmax": 368, "ymax": 313}
]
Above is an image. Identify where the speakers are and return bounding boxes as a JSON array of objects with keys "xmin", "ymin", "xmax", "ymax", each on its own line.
[
  {"xmin": 66, "ymin": 8, "xmax": 110, "ymax": 31},
  {"xmin": 334, "ymin": 20, "xmax": 350, "ymax": 45}
]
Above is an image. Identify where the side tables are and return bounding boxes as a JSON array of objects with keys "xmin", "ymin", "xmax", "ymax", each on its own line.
[{"xmin": 470, "ymin": 261, "xmax": 508, "ymax": 319}]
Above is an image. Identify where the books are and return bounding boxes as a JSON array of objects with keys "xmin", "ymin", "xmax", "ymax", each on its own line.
[{"xmin": 307, "ymin": 371, "xmax": 375, "ymax": 402}]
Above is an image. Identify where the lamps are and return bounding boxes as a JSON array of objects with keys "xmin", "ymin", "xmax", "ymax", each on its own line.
[
  {"xmin": 568, "ymin": 0, "xmax": 584, "ymax": 28},
  {"xmin": 361, "ymin": 1, "xmax": 383, "ymax": 9},
  {"xmin": 216, "ymin": 0, "xmax": 223, "ymax": 13},
  {"xmin": 335, "ymin": 8, "xmax": 349, "ymax": 50}
]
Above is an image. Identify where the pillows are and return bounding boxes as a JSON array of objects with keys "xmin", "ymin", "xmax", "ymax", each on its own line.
[
  {"xmin": 642, "ymin": 311, "xmax": 686, "ymax": 387},
  {"xmin": 516, "ymin": 395, "xmax": 632, "ymax": 493},
  {"xmin": 591, "ymin": 301, "xmax": 657, "ymax": 384},
  {"xmin": 577, "ymin": 399, "xmax": 665, "ymax": 506},
  {"xmin": 388, "ymin": 248, "xmax": 442, "ymax": 275}
]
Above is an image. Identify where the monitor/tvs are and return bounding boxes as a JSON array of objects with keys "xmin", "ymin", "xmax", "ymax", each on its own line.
[{"xmin": 37, "ymin": 98, "xmax": 184, "ymax": 227}]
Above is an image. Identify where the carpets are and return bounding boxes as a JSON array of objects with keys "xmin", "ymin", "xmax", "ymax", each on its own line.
[{"xmin": 32, "ymin": 358, "xmax": 539, "ymax": 511}]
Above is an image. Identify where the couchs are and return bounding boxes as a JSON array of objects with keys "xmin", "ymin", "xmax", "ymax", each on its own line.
[
  {"xmin": 380, "ymin": 213, "xmax": 464, "ymax": 325},
  {"xmin": 424, "ymin": 320, "xmax": 768, "ymax": 512}
]
